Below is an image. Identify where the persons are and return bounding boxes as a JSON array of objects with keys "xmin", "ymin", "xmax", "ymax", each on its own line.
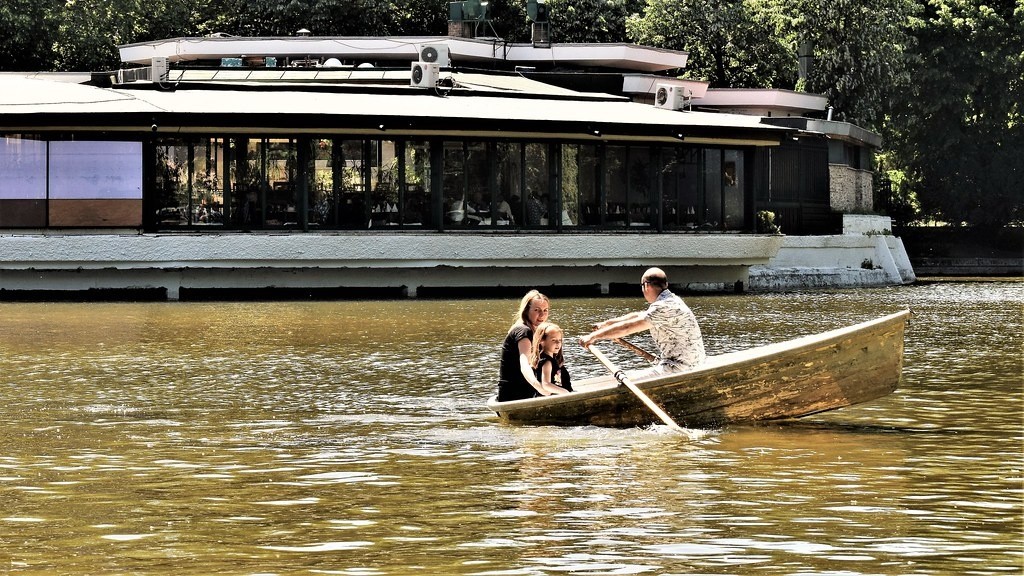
[
  {"xmin": 444, "ymin": 191, "xmax": 551, "ymax": 226},
  {"xmin": 498, "ymin": 290, "xmax": 572, "ymax": 402},
  {"xmin": 529, "ymin": 322, "xmax": 573, "ymax": 397},
  {"xmin": 663, "ymin": 195, "xmax": 672, "ymax": 230},
  {"xmin": 579, "ymin": 267, "xmax": 706, "ymax": 382},
  {"xmin": 230, "ymin": 172, "xmax": 433, "ymax": 226}
]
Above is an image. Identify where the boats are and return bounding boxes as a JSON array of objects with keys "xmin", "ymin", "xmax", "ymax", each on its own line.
[{"xmin": 485, "ymin": 307, "xmax": 910, "ymax": 430}]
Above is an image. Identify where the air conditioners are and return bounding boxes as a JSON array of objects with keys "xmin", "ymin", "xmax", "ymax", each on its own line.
[
  {"xmin": 419, "ymin": 43, "xmax": 449, "ymax": 65},
  {"xmin": 411, "ymin": 62, "xmax": 440, "ymax": 86},
  {"xmin": 654, "ymin": 84, "xmax": 684, "ymax": 110},
  {"xmin": 152, "ymin": 57, "xmax": 170, "ymax": 82}
]
